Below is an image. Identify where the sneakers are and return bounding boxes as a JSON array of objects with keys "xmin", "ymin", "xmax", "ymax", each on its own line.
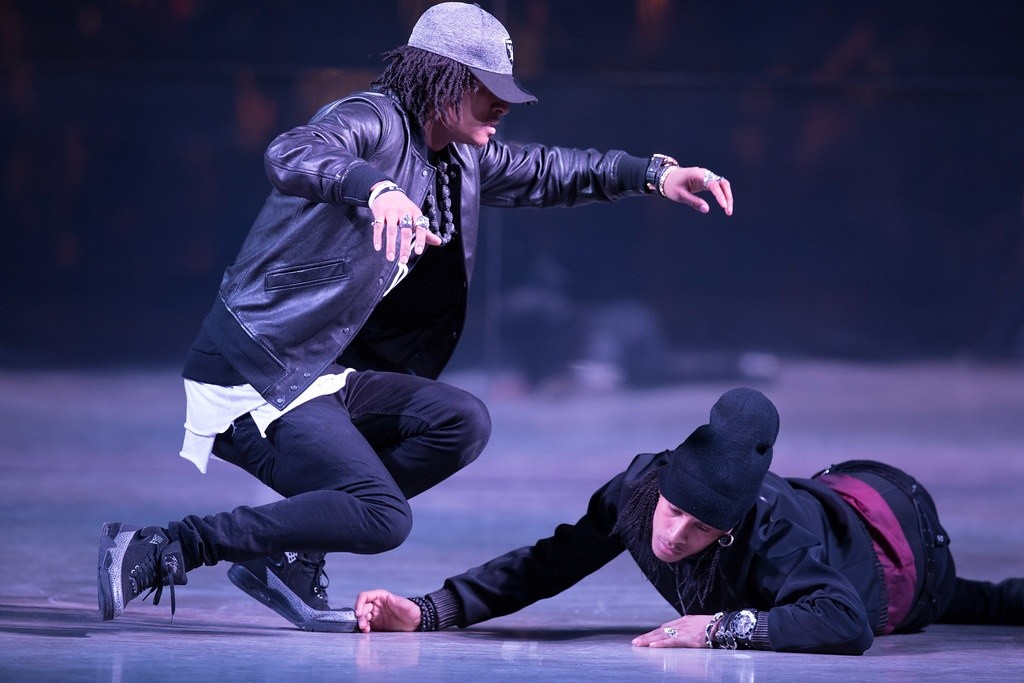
[
  {"xmin": 97, "ymin": 521, "xmax": 188, "ymax": 621},
  {"xmin": 227, "ymin": 554, "xmax": 358, "ymax": 632}
]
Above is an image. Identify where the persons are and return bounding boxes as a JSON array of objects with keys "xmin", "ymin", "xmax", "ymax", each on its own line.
[
  {"xmin": 97, "ymin": 0, "xmax": 735, "ymax": 635},
  {"xmin": 355, "ymin": 388, "xmax": 1024, "ymax": 656}
]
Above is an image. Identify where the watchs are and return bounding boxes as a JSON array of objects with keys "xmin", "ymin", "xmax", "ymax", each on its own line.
[
  {"xmin": 645, "ymin": 153, "xmax": 679, "ymax": 199},
  {"xmin": 729, "ymin": 608, "xmax": 758, "ymax": 649}
]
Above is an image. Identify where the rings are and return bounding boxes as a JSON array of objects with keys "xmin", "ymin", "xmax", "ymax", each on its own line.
[
  {"xmin": 703, "ymin": 171, "xmax": 717, "ymax": 187},
  {"xmin": 371, "ymin": 220, "xmax": 384, "ymax": 226},
  {"xmin": 714, "ymin": 175, "xmax": 722, "ymax": 181},
  {"xmin": 398, "ymin": 215, "xmax": 413, "ymax": 229},
  {"xmin": 414, "ymin": 216, "xmax": 430, "ymax": 232},
  {"xmin": 665, "ymin": 628, "xmax": 678, "ymax": 638}
]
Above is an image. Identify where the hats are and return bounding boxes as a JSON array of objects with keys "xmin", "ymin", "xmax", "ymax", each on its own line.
[
  {"xmin": 659, "ymin": 387, "xmax": 780, "ymax": 532},
  {"xmin": 408, "ymin": 2, "xmax": 539, "ymax": 106}
]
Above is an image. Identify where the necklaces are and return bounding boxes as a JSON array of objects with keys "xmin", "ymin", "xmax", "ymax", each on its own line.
[{"xmin": 424, "ymin": 161, "xmax": 457, "ymax": 248}]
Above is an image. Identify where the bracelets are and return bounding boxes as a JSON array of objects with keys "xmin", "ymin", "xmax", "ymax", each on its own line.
[
  {"xmin": 707, "ymin": 609, "xmax": 739, "ymax": 651},
  {"xmin": 656, "ymin": 164, "xmax": 678, "ymax": 199},
  {"xmin": 407, "ymin": 596, "xmax": 437, "ymax": 632}
]
